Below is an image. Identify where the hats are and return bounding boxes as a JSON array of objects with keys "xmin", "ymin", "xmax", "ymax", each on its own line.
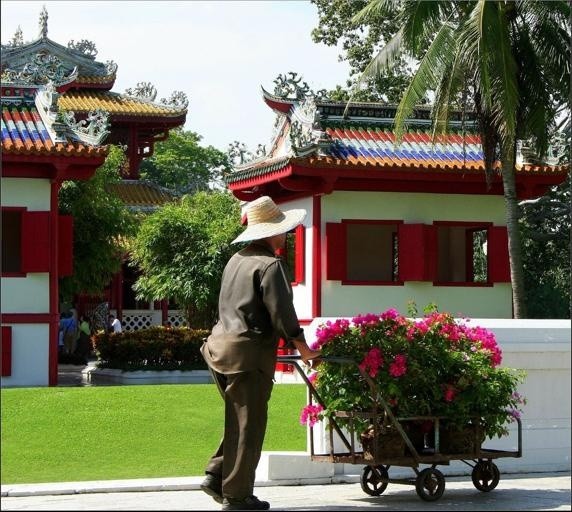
[{"xmin": 229, "ymin": 196, "xmax": 308, "ymax": 245}]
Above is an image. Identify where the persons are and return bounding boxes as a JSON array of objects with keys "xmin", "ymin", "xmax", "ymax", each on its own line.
[
  {"xmin": 199, "ymin": 196, "xmax": 323, "ymax": 511},
  {"xmin": 108, "ymin": 315, "xmax": 122, "ymax": 334},
  {"xmin": 58, "ymin": 312, "xmax": 91, "ymax": 365}
]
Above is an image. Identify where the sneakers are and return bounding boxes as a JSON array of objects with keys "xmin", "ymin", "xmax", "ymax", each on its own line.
[{"xmin": 200, "ymin": 471, "xmax": 270, "ymax": 510}]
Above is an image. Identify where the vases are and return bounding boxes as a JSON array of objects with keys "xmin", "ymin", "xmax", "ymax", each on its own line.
[{"xmin": 359, "ymin": 419, "xmax": 488, "ymax": 456}]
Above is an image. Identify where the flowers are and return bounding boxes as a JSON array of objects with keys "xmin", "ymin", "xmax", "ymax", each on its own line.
[{"xmin": 297, "ymin": 300, "xmax": 526, "ymax": 440}]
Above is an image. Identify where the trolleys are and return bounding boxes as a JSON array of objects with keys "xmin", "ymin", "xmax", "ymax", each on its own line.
[{"xmin": 276, "ymin": 355, "xmax": 522, "ymax": 502}]
